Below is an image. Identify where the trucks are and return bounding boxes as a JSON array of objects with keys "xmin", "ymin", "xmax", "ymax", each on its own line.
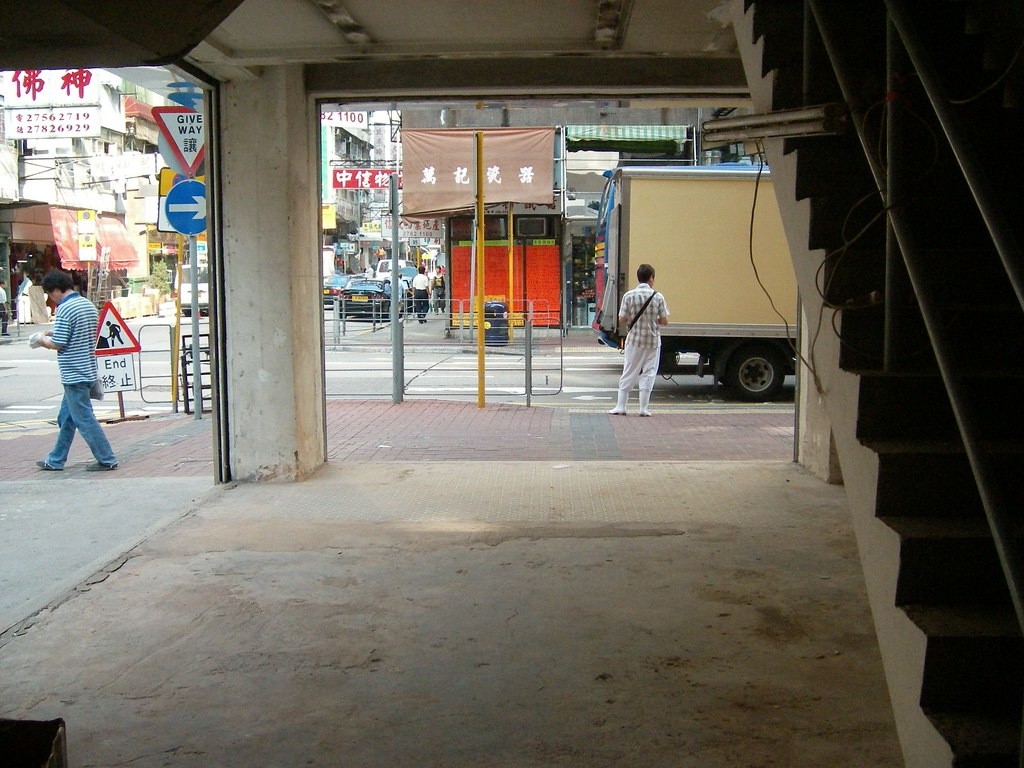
[{"xmin": 592, "ymin": 162, "xmax": 802, "ymax": 402}]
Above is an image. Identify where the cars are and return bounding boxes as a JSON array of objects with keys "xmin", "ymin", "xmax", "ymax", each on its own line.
[
  {"xmin": 384, "ymin": 266, "xmax": 417, "ymax": 293},
  {"xmin": 322, "ymin": 275, "xmax": 349, "ymax": 307},
  {"xmin": 340, "ymin": 278, "xmax": 405, "ymax": 320}
]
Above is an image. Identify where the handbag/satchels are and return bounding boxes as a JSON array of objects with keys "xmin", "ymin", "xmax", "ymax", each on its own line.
[{"xmin": 90, "ymin": 370, "xmax": 105, "ymax": 401}]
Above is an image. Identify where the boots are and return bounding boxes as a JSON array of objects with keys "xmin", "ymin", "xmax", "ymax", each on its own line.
[
  {"xmin": 639, "ymin": 390, "xmax": 651, "ymax": 416},
  {"xmin": 609, "ymin": 390, "xmax": 628, "ymax": 415}
]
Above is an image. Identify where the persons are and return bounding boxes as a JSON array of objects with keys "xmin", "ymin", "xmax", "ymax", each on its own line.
[
  {"xmin": 609, "ymin": 264, "xmax": 669, "ymax": 418},
  {"xmin": 35, "ymin": 270, "xmax": 119, "ymax": 471},
  {"xmin": 347, "ymin": 264, "xmax": 374, "ymax": 279},
  {"xmin": 388, "ymin": 265, "xmax": 445, "ymax": 329},
  {"xmin": 0, "ymin": 279, "xmax": 24, "ymax": 337}
]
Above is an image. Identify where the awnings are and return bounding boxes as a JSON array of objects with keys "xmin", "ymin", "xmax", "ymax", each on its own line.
[
  {"xmin": 125, "ymin": 96, "xmax": 158, "ymax": 125},
  {"xmin": 566, "ymin": 125, "xmax": 687, "ymax": 140},
  {"xmin": 48, "ymin": 207, "xmax": 140, "ymax": 272},
  {"xmin": 568, "ymin": 141, "xmax": 676, "ymax": 154}
]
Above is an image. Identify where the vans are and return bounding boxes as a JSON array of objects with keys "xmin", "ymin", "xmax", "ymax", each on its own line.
[
  {"xmin": 375, "ymin": 259, "xmax": 415, "ymax": 278},
  {"xmin": 173, "ymin": 264, "xmax": 209, "ymax": 316}
]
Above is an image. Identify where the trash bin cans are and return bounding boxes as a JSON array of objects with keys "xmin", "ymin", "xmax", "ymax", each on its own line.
[{"xmin": 483, "ymin": 301, "xmax": 510, "ymax": 346}]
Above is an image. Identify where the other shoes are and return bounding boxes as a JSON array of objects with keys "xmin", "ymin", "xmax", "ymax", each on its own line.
[
  {"xmin": 86, "ymin": 462, "xmax": 118, "ymax": 471},
  {"xmin": 419, "ymin": 321, "xmax": 423, "ymax": 324},
  {"xmin": 420, "ymin": 315, "xmax": 427, "ymax": 323},
  {"xmin": 36, "ymin": 461, "xmax": 63, "ymax": 471},
  {"xmin": 1, "ymin": 334, "xmax": 10, "ymax": 336}
]
